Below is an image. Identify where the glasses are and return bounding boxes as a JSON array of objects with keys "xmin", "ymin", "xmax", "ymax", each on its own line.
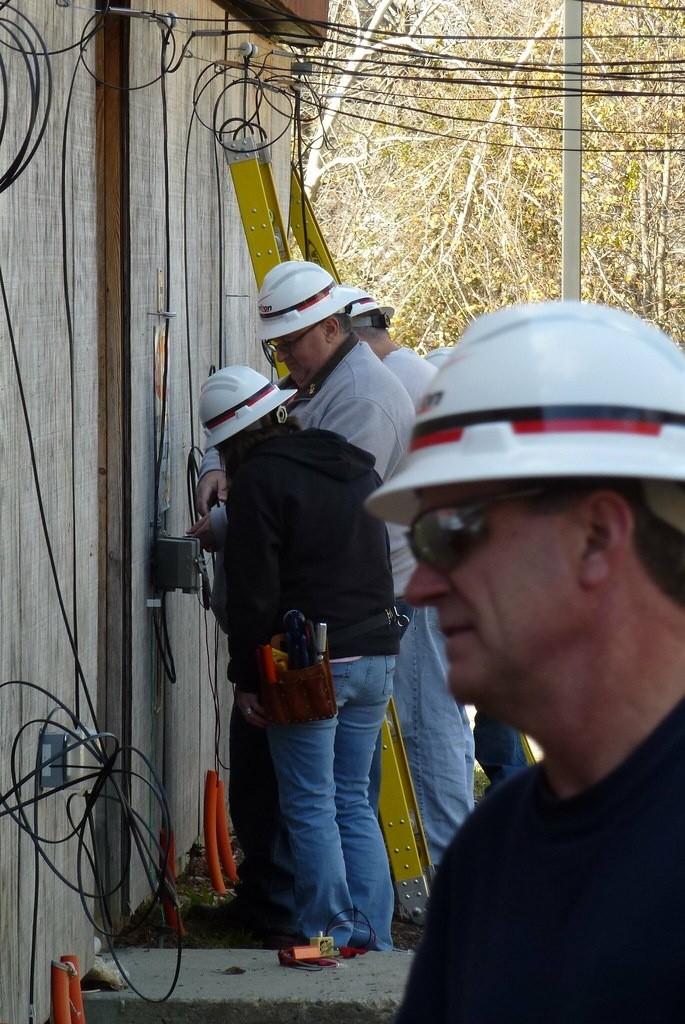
[
  {"xmin": 265, "ymin": 315, "xmax": 334, "ymax": 353},
  {"xmin": 408, "ymin": 484, "xmax": 547, "ymax": 572}
]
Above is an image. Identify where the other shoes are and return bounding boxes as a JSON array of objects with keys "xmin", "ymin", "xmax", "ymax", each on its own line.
[
  {"xmin": 262, "ymin": 934, "xmax": 305, "ymax": 949},
  {"xmin": 184, "ymin": 904, "xmax": 279, "ymax": 942}
]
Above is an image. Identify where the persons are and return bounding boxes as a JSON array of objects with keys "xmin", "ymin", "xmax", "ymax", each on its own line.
[
  {"xmin": 424, "ymin": 349, "xmax": 527, "ymax": 799},
  {"xmin": 365, "ymin": 301, "xmax": 685, "ymax": 1024},
  {"xmin": 186, "ymin": 367, "xmax": 399, "ymax": 958},
  {"xmin": 187, "ymin": 261, "xmax": 405, "ymax": 934},
  {"xmin": 329, "ymin": 286, "xmax": 475, "ymax": 865}
]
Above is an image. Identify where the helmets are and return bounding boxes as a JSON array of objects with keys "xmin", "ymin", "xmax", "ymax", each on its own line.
[
  {"xmin": 339, "ymin": 285, "xmax": 395, "ymax": 320},
  {"xmin": 255, "ymin": 261, "xmax": 352, "ymax": 339},
  {"xmin": 198, "ymin": 365, "xmax": 298, "ymax": 450},
  {"xmin": 363, "ymin": 302, "xmax": 685, "ymax": 526}
]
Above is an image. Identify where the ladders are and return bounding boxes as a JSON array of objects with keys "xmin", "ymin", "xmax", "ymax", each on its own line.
[
  {"xmin": 223, "ymin": 133, "xmax": 438, "ymax": 925},
  {"xmin": 287, "ymin": 159, "xmax": 538, "ymax": 777}
]
